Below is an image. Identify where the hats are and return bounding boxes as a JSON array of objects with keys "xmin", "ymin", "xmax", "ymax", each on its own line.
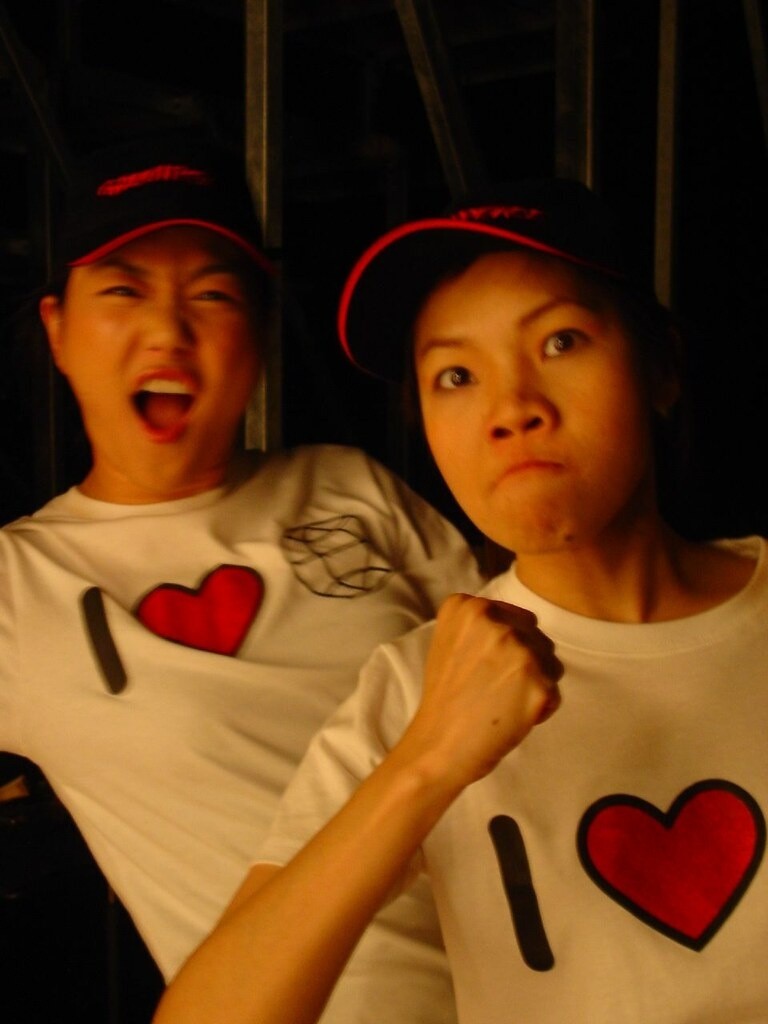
[
  {"xmin": 340, "ymin": 174, "xmax": 664, "ymax": 372},
  {"xmin": 52, "ymin": 157, "xmax": 261, "ymax": 281}
]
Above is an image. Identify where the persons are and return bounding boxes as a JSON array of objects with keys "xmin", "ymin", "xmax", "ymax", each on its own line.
[
  {"xmin": 148, "ymin": 186, "xmax": 768, "ymax": 1023},
  {"xmin": 0, "ymin": 147, "xmax": 486, "ymax": 1024}
]
